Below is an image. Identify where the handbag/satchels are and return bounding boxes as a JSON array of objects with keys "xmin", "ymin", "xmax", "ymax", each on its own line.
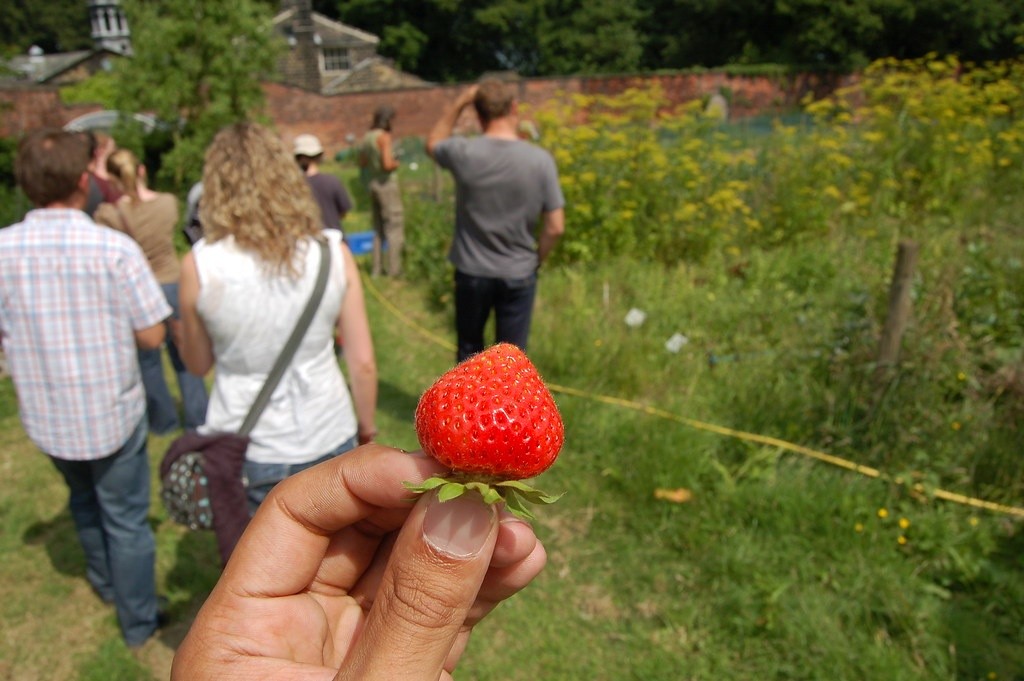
[{"xmin": 158, "ymin": 431, "xmax": 251, "ymax": 530}]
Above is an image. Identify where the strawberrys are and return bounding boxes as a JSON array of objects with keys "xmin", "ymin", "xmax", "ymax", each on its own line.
[{"xmin": 414, "ymin": 341, "xmax": 565, "ymax": 479}]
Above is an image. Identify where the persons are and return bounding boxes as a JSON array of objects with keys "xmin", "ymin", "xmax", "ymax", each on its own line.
[
  {"xmin": 426, "ymin": 72, "xmax": 567, "ymax": 366},
  {"xmin": 170, "ymin": 443, "xmax": 548, "ymax": 681},
  {"xmin": 358, "ymin": 104, "xmax": 405, "ymax": 278},
  {"xmin": 93, "ymin": 149, "xmax": 209, "ymax": 437},
  {"xmin": 293, "ymin": 133, "xmax": 352, "ymax": 239},
  {"xmin": 0, "ymin": 124, "xmax": 209, "ymax": 650},
  {"xmin": 179, "ymin": 123, "xmax": 378, "ymax": 526}
]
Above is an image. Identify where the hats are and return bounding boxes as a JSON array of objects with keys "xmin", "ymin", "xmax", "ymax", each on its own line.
[{"xmin": 292, "ymin": 134, "xmax": 323, "ymax": 157}]
